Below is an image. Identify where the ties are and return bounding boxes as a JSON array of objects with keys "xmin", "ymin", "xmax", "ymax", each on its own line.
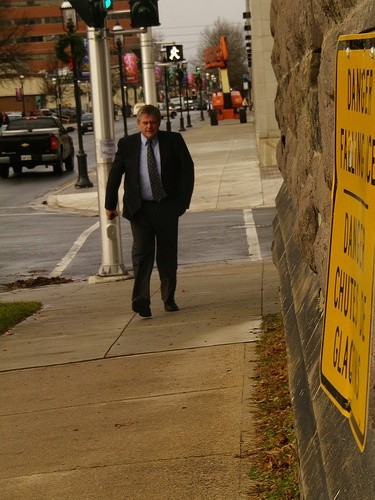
[{"xmin": 147, "ymin": 138, "xmax": 166, "ymax": 205}]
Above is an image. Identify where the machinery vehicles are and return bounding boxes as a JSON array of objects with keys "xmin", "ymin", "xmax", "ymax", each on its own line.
[{"xmin": 206, "ymin": 35, "xmax": 247, "ymax": 126}]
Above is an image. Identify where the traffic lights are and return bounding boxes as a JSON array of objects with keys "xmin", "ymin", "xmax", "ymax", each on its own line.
[
  {"xmin": 67, "ymin": 0, "xmax": 104, "ymax": 28},
  {"xmin": 165, "ymin": 44, "xmax": 184, "ymax": 62},
  {"xmin": 131, "ymin": 48, "xmax": 142, "ymax": 71},
  {"xmin": 102, "ymin": 0, "xmax": 114, "ymax": 10},
  {"xmin": 128, "ymin": 0, "xmax": 162, "ymax": 29}
]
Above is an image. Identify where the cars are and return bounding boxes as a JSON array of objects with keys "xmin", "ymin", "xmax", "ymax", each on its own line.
[
  {"xmin": 132, "ymin": 98, "xmax": 208, "ymax": 120},
  {"xmin": 0, "ymin": 108, "xmax": 79, "ymax": 180},
  {"xmin": 79, "ymin": 113, "xmax": 94, "ymax": 136}
]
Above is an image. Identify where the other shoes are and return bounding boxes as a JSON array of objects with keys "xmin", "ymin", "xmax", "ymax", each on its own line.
[
  {"xmin": 132, "ymin": 299, "xmax": 152, "ymax": 317},
  {"xmin": 163, "ymin": 299, "xmax": 178, "ymax": 311}
]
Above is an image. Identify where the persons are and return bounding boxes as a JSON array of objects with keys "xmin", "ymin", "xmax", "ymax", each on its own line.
[
  {"xmin": 104, "ymin": 104, "xmax": 195, "ymax": 317},
  {"xmin": 242, "ymin": 98, "xmax": 248, "ymax": 111}
]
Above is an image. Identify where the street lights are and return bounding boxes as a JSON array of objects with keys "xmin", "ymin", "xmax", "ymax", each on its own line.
[
  {"xmin": 51, "ymin": 74, "xmax": 58, "ymax": 110},
  {"xmin": 59, "ymin": 2, "xmax": 94, "ymax": 188},
  {"xmin": 112, "ymin": 19, "xmax": 129, "ymax": 136},
  {"xmin": 160, "ymin": 44, "xmax": 172, "ymax": 132},
  {"xmin": 19, "ymin": 72, "xmax": 26, "ymax": 117},
  {"xmin": 178, "ymin": 59, "xmax": 216, "ymax": 131}
]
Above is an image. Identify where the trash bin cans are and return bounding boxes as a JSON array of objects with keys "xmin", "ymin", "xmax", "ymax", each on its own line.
[
  {"xmin": 41, "ymin": 109, "xmax": 49, "ymax": 116},
  {"xmin": 239, "ymin": 107, "xmax": 246, "ymax": 123},
  {"xmin": 211, "ymin": 109, "xmax": 218, "ymax": 125},
  {"xmin": 122, "ymin": 105, "xmax": 131, "ymax": 115}
]
